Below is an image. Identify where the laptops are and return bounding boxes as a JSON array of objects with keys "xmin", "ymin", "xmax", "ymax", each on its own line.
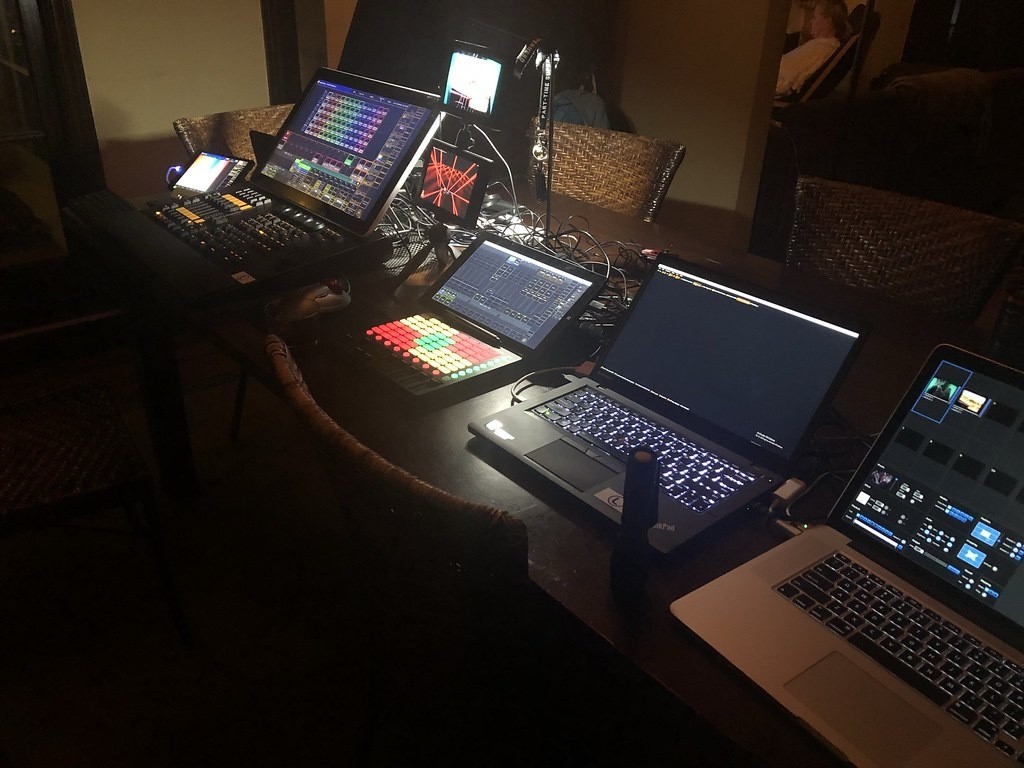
[
  {"xmin": 669, "ymin": 344, "xmax": 1024, "ymax": 768},
  {"xmin": 466, "ymin": 251, "xmax": 872, "ymax": 556}
]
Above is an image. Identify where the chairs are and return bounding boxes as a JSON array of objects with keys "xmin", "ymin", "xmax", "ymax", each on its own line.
[
  {"xmin": 0, "ymin": 371, "xmax": 200, "ymax": 649},
  {"xmin": 782, "ymin": 67, "xmax": 1024, "ymax": 215},
  {"xmin": 265, "ymin": 334, "xmax": 619, "ymax": 768},
  {"xmin": 789, "ymin": 2, "xmax": 882, "ymax": 102},
  {"xmin": 173, "ymin": 103, "xmax": 295, "ymax": 178},
  {"xmin": 528, "ymin": 117, "xmax": 688, "ymax": 224},
  {"xmin": 786, "ymin": 173, "xmax": 1024, "ymax": 333}
]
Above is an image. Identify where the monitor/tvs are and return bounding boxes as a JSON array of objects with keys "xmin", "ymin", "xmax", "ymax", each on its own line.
[
  {"xmin": 440, "ymin": 39, "xmax": 516, "ymax": 132},
  {"xmin": 248, "ymin": 67, "xmax": 447, "ymax": 242},
  {"xmin": 168, "ymin": 149, "xmax": 255, "ymax": 196},
  {"xmin": 413, "ymin": 138, "xmax": 495, "ymax": 229},
  {"xmin": 418, "ymin": 233, "xmax": 608, "ymax": 365}
]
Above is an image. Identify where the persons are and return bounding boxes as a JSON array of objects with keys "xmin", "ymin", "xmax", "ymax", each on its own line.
[{"xmin": 774, "ymin": 0, "xmax": 851, "ymax": 102}]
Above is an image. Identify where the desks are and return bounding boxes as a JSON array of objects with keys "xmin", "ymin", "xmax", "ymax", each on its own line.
[{"xmin": 238, "ymin": 173, "xmax": 1024, "ymax": 768}]
